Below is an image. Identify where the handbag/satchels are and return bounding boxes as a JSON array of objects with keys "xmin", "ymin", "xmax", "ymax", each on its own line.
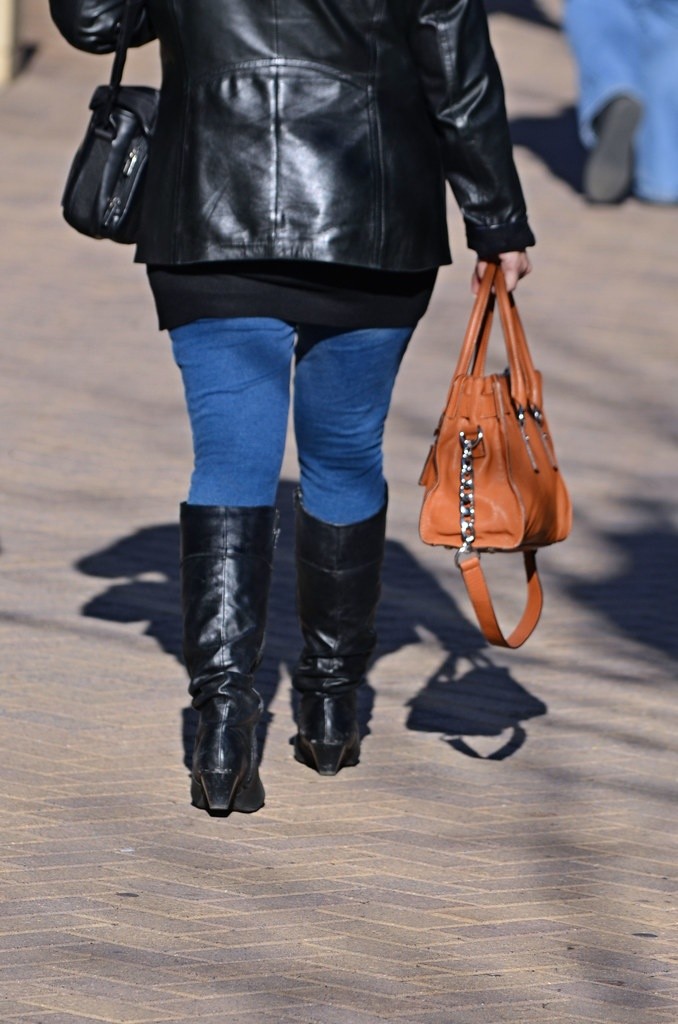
[
  {"xmin": 61, "ymin": 0, "xmax": 164, "ymax": 245},
  {"xmin": 415, "ymin": 263, "xmax": 572, "ymax": 651}
]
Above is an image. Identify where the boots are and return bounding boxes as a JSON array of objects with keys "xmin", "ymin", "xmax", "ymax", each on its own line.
[
  {"xmin": 294, "ymin": 479, "xmax": 389, "ymax": 773},
  {"xmin": 178, "ymin": 501, "xmax": 278, "ymax": 812}
]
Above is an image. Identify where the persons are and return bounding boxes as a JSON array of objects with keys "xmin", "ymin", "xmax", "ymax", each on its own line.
[
  {"xmin": 562, "ymin": 1, "xmax": 676, "ymax": 211},
  {"xmin": 49, "ymin": 1, "xmax": 537, "ymax": 818}
]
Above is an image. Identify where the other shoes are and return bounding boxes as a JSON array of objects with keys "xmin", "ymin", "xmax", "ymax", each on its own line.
[{"xmin": 582, "ymin": 96, "xmax": 641, "ymax": 204}]
